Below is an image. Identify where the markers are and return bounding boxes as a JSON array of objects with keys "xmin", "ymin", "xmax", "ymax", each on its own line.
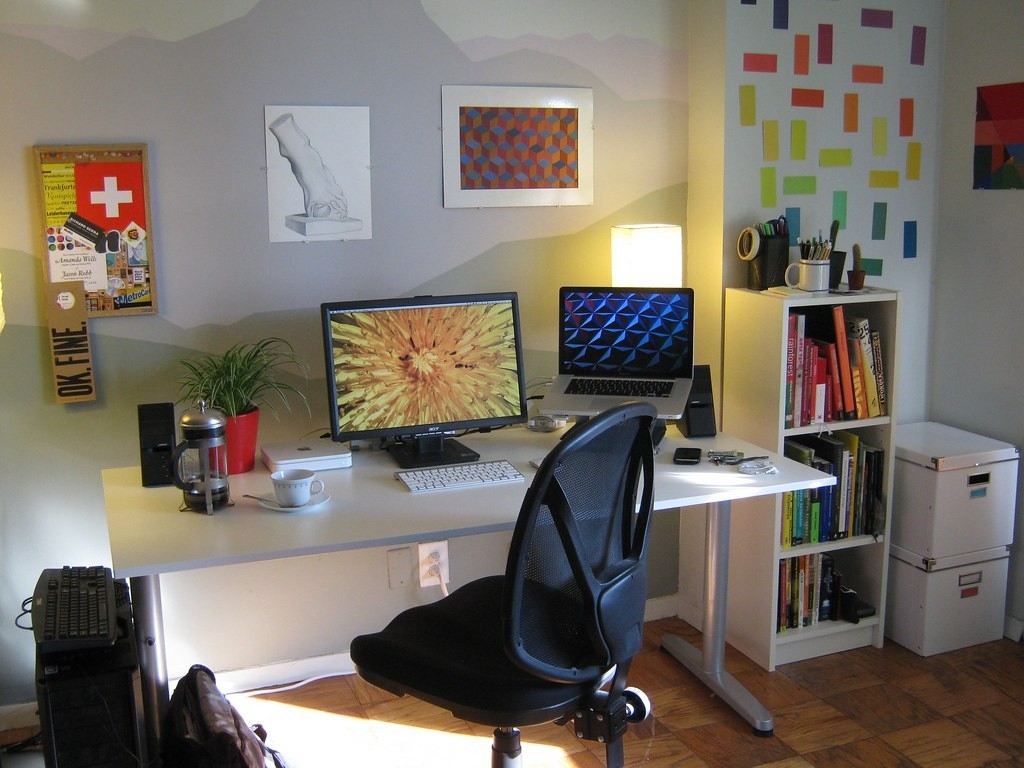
[{"xmin": 777, "ymin": 215, "xmax": 786, "ymax": 235}]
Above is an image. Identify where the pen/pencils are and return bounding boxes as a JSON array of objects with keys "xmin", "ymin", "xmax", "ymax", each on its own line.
[
  {"xmin": 796, "ymin": 237, "xmax": 832, "ymax": 261},
  {"xmin": 819, "ymin": 229, "xmax": 822, "ymax": 242},
  {"xmin": 754, "ymin": 222, "xmax": 776, "ymax": 235}
]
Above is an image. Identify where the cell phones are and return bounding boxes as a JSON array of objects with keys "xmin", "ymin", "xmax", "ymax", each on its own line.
[{"xmin": 673, "ymin": 447, "xmax": 702, "ymax": 464}]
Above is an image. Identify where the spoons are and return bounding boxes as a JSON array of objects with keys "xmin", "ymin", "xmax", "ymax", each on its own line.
[{"xmin": 243, "ymin": 495, "xmax": 300, "ymax": 508}]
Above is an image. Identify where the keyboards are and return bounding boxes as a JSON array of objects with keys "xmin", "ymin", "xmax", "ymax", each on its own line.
[
  {"xmin": 29, "ymin": 566, "xmax": 117, "ymax": 650},
  {"xmin": 393, "ymin": 459, "xmax": 525, "ymax": 493}
]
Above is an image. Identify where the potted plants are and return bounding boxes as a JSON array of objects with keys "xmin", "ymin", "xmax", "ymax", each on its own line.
[
  {"xmin": 829, "ymin": 220, "xmax": 847, "ymax": 288},
  {"xmin": 174, "ymin": 335, "xmax": 312, "ymax": 475},
  {"xmin": 847, "ymin": 244, "xmax": 866, "ymax": 290}
]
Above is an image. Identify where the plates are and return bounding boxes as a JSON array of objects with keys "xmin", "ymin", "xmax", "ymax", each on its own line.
[{"xmin": 259, "ymin": 492, "xmax": 331, "ymax": 512}]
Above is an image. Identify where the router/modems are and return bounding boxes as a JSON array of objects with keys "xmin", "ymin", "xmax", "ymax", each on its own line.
[{"xmin": 259, "ymin": 438, "xmax": 352, "ymax": 475}]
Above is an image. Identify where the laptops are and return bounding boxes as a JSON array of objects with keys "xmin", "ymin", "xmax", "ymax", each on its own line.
[{"xmin": 538, "ymin": 286, "xmax": 695, "ymax": 420}]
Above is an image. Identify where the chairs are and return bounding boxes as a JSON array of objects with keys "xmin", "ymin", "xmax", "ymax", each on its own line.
[{"xmin": 350, "ymin": 401, "xmax": 658, "ymax": 768}]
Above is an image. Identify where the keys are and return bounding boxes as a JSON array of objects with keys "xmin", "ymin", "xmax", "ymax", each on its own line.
[{"xmin": 710, "ymin": 456, "xmax": 723, "ymax": 466}]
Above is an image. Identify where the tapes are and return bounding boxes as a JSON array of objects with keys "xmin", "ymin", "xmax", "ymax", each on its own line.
[{"xmin": 736, "ymin": 227, "xmax": 760, "ymax": 260}]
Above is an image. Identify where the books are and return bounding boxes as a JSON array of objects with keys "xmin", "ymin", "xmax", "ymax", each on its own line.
[
  {"xmin": 784, "ymin": 304, "xmax": 888, "ymax": 429},
  {"xmin": 781, "ymin": 430, "xmax": 885, "ymax": 551},
  {"xmin": 776, "ymin": 552, "xmax": 876, "ymax": 634}
]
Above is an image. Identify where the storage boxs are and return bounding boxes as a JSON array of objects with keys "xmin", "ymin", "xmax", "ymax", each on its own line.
[
  {"xmin": 890, "ymin": 421, "xmax": 1020, "ymax": 560},
  {"xmin": 884, "ymin": 542, "xmax": 1010, "ymax": 658}
]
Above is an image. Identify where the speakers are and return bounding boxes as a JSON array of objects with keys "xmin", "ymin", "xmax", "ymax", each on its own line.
[
  {"xmin": 676, "ymin": 365, "xmax": 718, "ymax": 439},
  {"xmin": 137, "ymin": 402, "xmax": 177, "ymax": 488}
]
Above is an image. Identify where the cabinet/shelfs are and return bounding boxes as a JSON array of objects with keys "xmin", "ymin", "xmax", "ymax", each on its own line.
[{"xmin": 720, "ymin": 286, "xmax": 902, "ymax": 671}]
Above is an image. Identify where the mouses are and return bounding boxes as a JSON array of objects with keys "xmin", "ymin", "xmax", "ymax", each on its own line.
[{"xmin": 528, "ymin": 459, "xmax": 543, "ymax": 468}]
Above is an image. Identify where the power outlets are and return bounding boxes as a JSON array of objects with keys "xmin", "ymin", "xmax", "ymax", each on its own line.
[{"xmin": 417, "ymin": 539, "xmax": 450, "ymax": 588}]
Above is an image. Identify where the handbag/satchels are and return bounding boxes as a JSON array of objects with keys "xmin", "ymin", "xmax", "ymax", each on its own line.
[{"xmin": 159, "ymin": 664, "xmax": 285, "ymax": 768}]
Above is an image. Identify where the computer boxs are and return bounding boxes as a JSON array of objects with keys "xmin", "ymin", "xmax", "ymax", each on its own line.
[{"xmin": 34, "ymin": 577, "xmax": 151, "ymax": 768}]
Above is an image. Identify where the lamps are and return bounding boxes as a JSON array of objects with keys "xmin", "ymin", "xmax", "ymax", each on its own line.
[{"xmin": 611, "ymin": 223, "xmax": 683, "ymax": 289}]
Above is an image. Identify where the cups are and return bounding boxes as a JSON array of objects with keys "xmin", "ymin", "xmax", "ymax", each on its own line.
[
  {"xmin": 269, "ymin": 469, "xmax": 324, "ymax": 507},
  {"xmin": 746, "ymin": 235, "xmax": 790, "ymax": 292},
  {"xmin": 169, "ymin": 400, "xmax": 235, "ymax": 516},
  {"xmin": 785, "ymin": 260, "xmax": 830, "ymax": 291}
]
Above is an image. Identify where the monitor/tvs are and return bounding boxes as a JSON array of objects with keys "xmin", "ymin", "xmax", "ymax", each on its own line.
[{"xmin": 321, "ymin": 291, "xmax": 529, "ymax": 470}]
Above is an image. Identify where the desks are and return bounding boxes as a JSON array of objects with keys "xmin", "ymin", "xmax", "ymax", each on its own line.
[{"xmin": 101, "ymin": 416, "xmax": 837, "ymax": 767}]
